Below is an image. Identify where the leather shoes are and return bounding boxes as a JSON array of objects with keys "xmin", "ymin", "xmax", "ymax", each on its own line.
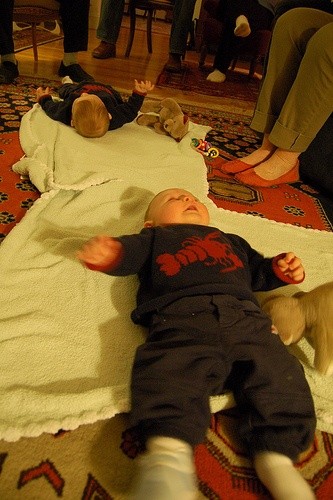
[
  {"xmin": 92, "ymin": 41, "xmax": 116, "ymax": 58},
  {"xmin": 165, "ymin": 54, "xmax": 183, "ymax": 72}
]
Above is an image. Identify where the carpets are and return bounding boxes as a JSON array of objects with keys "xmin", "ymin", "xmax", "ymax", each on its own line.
[
  {"xmin": 157, "ymin": 61, "xmax": 263, "ymax": 100},
  {"xmin": 0, "ymin": 72, "xmax": 333, "ymax": 500},
  {"xmin": 13, "ymin": 26, "xmax": 64, "ymax": 52}
]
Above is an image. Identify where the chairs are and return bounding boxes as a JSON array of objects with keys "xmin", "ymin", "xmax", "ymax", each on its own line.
[
  {"xmin": 196, "ymin": 0, "xmax": 272, "ymax": 82},
  {"xmin": 13, "ymin": 0, "xmax": 60, "ymax": 61},
  {"xmin": 124, "ymin": 0, "xmax": 196, "ymax": 58}
]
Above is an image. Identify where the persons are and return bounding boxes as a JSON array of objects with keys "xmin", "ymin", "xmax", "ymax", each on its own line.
[
  {"xmin": 206, "ymin": 0, "xmax": 283, "ymax": 83},
  {"xmin": 36, "ymin": 75, "xmax": 155, "ymax": 138},
  {"xmin": 0, "ymin": 0, "xmax": 96, "ymax": 85},
  {"xmin": 220, "ymin": 7, "xmax": 333, "ymax": 188},
  {"xmin": 72, "ymin": 187, "xmax": 316, "ymax": 500},
  {"xmin": 91, "ymin": 0, "xmax": 197, "ymax": 73}
]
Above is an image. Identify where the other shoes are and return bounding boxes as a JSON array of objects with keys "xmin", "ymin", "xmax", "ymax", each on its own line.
[
  {"xmin": 58, "ymin": 60, "xmax": 95, "ymax": 83},
  {"xmin": 220, "ymin": 147, "xmax": 299, "ymax": 186},
  {"xmin": 0, "ymin": 60, "xmax": 19, "ymax": 84}
]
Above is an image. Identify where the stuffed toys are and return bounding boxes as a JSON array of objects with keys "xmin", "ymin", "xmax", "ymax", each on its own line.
[
  {"xmin": 260, "ymin": 281, "xmax": 333, "ymax": 376},
  {"xmin": 136, "ymin": 97, "xmax": 190, "ymax": 143}
]
